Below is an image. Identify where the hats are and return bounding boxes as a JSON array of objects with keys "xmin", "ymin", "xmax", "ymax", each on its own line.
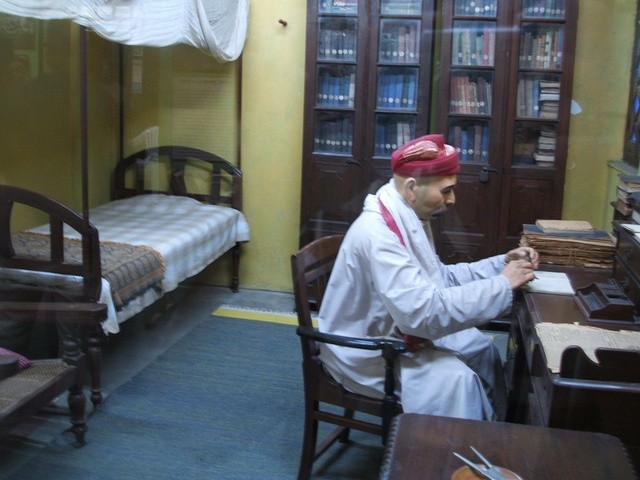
[{"xmin": 391, "ymin": 133, "xmax": 461, "ymax": 177}]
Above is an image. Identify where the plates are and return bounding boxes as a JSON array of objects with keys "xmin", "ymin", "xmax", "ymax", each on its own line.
[{"xmin": 451, "ymin": 463, "xmax": 523, "ymax": 480}]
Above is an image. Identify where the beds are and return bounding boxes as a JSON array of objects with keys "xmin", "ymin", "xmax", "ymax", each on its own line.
[{"xmin": 1, "ymin": 1, "xmax": 250, "ymax": 408}]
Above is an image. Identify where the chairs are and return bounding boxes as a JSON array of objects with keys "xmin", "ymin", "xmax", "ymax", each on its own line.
[
  {"xmin": 0, "ymin": 302, "xmax": 108, "ymax": 463},
  {"xmin": 290, "ymin": 233, "xmax": 409, "ymax": 479}
]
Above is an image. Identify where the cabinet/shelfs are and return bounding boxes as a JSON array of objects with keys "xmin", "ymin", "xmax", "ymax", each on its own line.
[
  {"xmin": 294, "ymin": 0, "xmax": 435, "ymax": 313},
  {"xmin": 502, "ymin": 202, "xmax": 640, "ymax": 429},
  {"xmin": 434, "ymin": 0, "xmax": 579, "ymax": 329}
]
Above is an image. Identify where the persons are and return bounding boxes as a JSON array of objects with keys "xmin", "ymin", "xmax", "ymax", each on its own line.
[{"xmin": 318, "ymin": 133, "xmax": 540, "ymax": 421}]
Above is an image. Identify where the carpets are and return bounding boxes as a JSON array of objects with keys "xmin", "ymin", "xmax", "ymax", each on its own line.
[{"xmin": 11, "ymin": 287, "xmax": 511, "ymax": 479}]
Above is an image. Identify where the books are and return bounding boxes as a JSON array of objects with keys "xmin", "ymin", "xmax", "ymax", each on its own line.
[
  {"xmin": 454, "ymin": 127, "xmax": 461, "ymax": 151},
  {"xmin": 402, "ymin": 74, "xmax": 409, "ymax": 107},
  {"xmin": 408, "ymin": 75, "xmax": 416, "ymax": 107},
  {"xmin": 467, "ymin": 127, "xmax": 474, "ymax": 162},
  {"xmin": 387, "ymin": 75, "xmax": 396, "ymax": 107},
  {"xmin": 535, "ymin": 123, "xmax": 558, "ymax": 169},
  {"xmin": 481, "ymin": 127, "xmax": 489, "ymax": 164},
  {"xmin": 448, "ymin": 127, "xmax": 454, "ymax": 146},
  {"xmin": 404, "ymin": 124, "xmax": 410, "ymax": 145},
  {"xmin": 474, "ymin": 126, "xmax": 481, "ymax": 163},
  {"xmin": 394, "ymin": 75, "xmax": 403, "ymax": 107},
  {"xmin": 378, "ymin": 74, "xmax": 387, "ymax": 108},
  {"xmin": 381, "ymin": 126, "xmax": 386, "ymax": 156},
  {"xmin": 374, "ymin": 124, "xmax": 380, "ymax": 156},
  {"xmin": 376, "ymin": 1, "xmax": 421, "ymax": 67},
  {"xmin": 387, "ymin": 123, "xmax": 391, "ymax": 157},
  {"xmin": 398, "ymin": 124, "xmax": 402, "ymax": 148},
  {"xmin": 313, "ymin": 0, "xmax": 355, "ymax": 155},
  {"xmin": 519, "ymin": 159, "xmax": 639, "ymax": 269},
  {"xmin": 461, "ymin": 131, "xmax": 467, "ymax": 163},
  {"xmin": 450, "ymin": 1, "xmax": 568, "ymax": 119},
  {"xmin": 392, "ymin": 123, "xmax": 396, "ymax": 152}
]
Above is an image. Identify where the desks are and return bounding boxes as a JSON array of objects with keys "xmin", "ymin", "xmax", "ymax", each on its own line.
[{"xmin": 381, "ymin": 412, "xmax": 635, "ymax": 479}]
